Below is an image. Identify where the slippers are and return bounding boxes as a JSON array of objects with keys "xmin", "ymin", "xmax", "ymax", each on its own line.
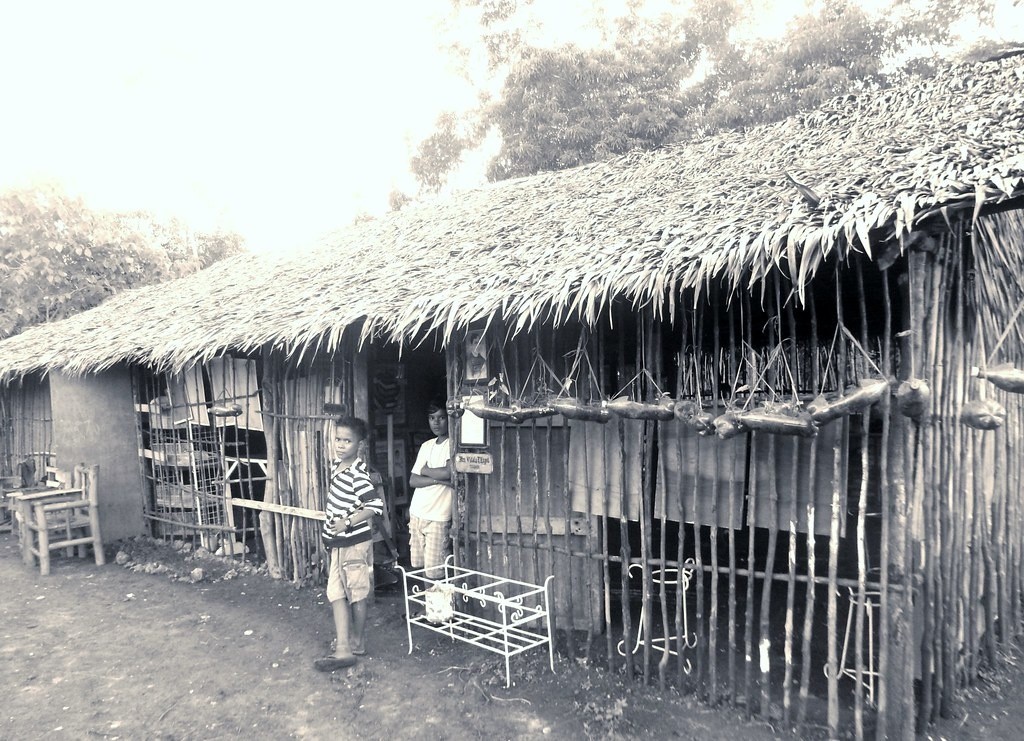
[
  {"xmin": 314, "ymin": 654, "xmax": 357, "ymax": 672},
  {"xmin": 331, "ymin": 637, "xmax": 366, "ymax": 655}
]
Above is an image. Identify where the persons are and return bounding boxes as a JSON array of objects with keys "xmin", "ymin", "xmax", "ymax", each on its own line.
[
  {"xmin": 312, "ymin": 414, "xmax": 385, "ymax": 671},
  {"xmin": 467, "ymin": 332, "xmax": 486, "ymax": 379},
  {"xmin": 400, "ymin": 396, "xmax": 451, "ymax": 629}
]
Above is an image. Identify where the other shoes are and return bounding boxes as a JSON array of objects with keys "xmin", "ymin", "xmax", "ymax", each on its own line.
[{"xmin": 402, "ymin": 611, "xmax": 420, "ymax": 621}]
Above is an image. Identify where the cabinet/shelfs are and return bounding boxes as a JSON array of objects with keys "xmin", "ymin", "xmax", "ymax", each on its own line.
[{"xmin": 392, "ymin": 554, "xmax": 558, "ymax": 691}]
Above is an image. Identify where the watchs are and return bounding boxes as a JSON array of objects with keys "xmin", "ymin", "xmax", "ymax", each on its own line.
[{"xmin": 343, "ymin": 518, "xmax": 352, "ymax": 531}]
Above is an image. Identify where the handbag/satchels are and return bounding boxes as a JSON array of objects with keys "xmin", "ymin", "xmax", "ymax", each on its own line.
[{"xmin": 424, "ymin": 582, "xmax": 455, "ymax": 624}]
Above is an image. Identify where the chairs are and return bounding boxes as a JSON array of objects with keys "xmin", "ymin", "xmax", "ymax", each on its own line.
[{"xmin": 0, "ymin": 457, "xmax": 105, "ymax": 575}]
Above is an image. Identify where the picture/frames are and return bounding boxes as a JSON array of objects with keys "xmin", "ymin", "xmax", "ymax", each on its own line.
[
  {"xmin": 460, "ymin": 324, "xmax": 491, "ymax": 386},
  {"xmin": 374, "ymin": 436, "xmax": 409, "ymax": 508},
  {"xmin": 370, "ymin": 357, "xmax": 408, "ymax": 429},
  {"xmin": 456, "ymin": 386, "xmax": 490, "ymax": 449}
]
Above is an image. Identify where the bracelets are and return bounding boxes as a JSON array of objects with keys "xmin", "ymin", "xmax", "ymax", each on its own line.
[{"xmin": 434, "ymin": 480, "xmax": 437, "ymax": 484}]
